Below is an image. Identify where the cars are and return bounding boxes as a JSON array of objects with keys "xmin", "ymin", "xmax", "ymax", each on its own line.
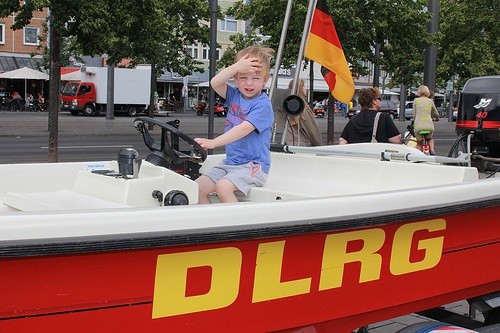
[
  {"xmin": 452, "ymin": 108, "xmax": 457, "ymax": 120},
  {"xmin": 311, "ymin": 105, "xmax": 325, "ymax": 118}
]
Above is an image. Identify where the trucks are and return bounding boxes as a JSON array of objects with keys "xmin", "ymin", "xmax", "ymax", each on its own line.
[{"xmin": 60, "ymin": 62, "xmax": 153, "ymax": 117}]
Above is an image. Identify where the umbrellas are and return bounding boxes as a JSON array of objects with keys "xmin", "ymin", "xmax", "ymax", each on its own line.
[
  {"xmin": 181, "ymin": 74, "xmax": 188, "ymax": 112},
  {"xmin": 0, "ymin": 68, "xmax": 50, "ymax": 101}
]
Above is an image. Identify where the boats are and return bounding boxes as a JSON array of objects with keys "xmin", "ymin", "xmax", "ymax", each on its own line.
[{"xmin": 1, "ymin": 73, "xmax": 497, "ymax": 332}]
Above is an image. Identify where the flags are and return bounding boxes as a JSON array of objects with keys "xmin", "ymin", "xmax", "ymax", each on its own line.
[{"xmin": 304, "ymin": 0, "xmax": 355, "ymax": 104}]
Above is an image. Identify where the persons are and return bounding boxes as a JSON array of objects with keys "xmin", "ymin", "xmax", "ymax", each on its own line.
[
  {"xmin": 165, "ymin": 87, "xmax": 208, "ymax": 109},
  {"xmin": 191, "ymin": 45, "xmax": 276, "ymax": 204},
  {"xmin": 11, "ymin": 90, "xmax": 48, "ymax": 111},
  {"xmin": 283, "ymin": 78, "xmax": 459, "ymax": 157}
]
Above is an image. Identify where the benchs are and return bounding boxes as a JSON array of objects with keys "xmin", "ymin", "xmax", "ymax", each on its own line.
[{"xmin": 193, "ymin": 152, "xmax": 479, "ymax": 202}]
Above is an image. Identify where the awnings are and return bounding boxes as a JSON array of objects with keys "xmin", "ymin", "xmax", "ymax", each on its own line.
[{"xmin": 190, "ymin": 79, "xmax": 445, "ymax": 99}]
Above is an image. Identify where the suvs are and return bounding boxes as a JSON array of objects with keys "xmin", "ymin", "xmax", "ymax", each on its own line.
[
  {"xmin": 397, "ymin": 100, "xmax": 414, "ymax": 119},
  {"xmin": 345, "ymin": 98, "xmax": 399, "ymax": 121}
]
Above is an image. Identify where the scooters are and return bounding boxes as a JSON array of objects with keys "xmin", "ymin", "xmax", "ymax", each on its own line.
[{"xmin": 194, "ymin": 97, "xmax": 225, "ymax": 119}]
